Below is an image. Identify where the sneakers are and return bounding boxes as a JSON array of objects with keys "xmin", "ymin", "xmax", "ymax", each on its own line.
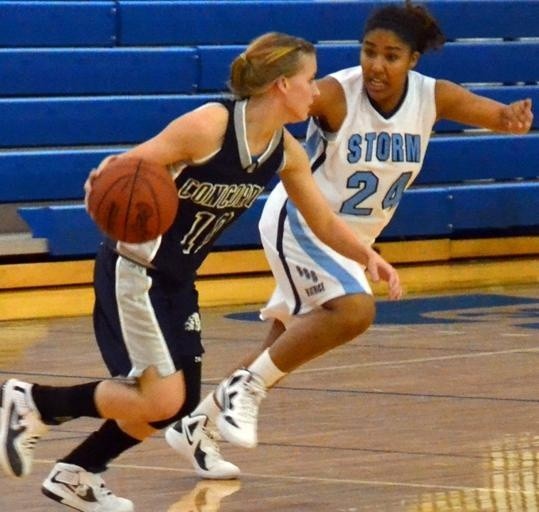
[
  {"xmin": 210, "ymin": 368, "xmax": 267, "ymax": 449},
  {"xmin": 165, "ymin": 413, "xmax": 240, "ymax": 479},
  {"xmin": 0, "ymin": 379, "xmax": 58, "ymax": 479},
  {"xmin": 41, "ymin": 460, "xmax": 135, "ymax": 512}
]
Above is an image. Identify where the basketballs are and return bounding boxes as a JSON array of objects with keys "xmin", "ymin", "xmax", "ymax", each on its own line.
[{"xmin": 87, "ymin": 154, "xmax": 180, "ymax": 243}]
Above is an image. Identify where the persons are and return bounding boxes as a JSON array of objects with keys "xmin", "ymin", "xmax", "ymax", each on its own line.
[
  {"xmin": 0, "ymin": 29, "xmax": 404, "ymax": 511},
  {"xmin": 162, "ymin": 5, "xmax": 533, "ymax": 480}
]
